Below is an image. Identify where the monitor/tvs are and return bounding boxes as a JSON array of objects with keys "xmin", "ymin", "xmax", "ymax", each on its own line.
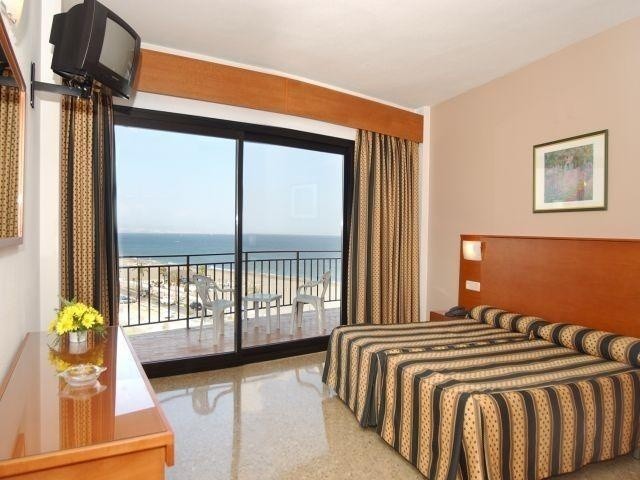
[{"xmin": 49, "ymin": 0, "xmax": 141, "ymax": 100}]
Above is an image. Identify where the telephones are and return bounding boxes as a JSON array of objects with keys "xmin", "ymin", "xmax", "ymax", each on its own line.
[{"xmin": 445, "ymin": 306, "xmax": 467, "ymax": 317}]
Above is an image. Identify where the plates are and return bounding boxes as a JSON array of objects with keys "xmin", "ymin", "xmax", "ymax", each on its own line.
[
  {"xmin": 56, "ymin": 380, "xmax": 107, "ymax": 400},
  {"xmin": 57, "ymin": 366, "xmax": 107, "ymax": 387}
]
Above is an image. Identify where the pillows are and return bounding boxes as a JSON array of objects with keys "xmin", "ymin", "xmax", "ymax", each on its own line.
[{"xmin": 470, "ymin": 305, "xmax": 640, "ymax": 368}]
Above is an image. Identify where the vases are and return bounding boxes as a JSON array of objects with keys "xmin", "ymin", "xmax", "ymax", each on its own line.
[
  {"xmin": 68, "ymin": 330, "xmax": 87, "ymax": 342},
  {"xmin": 68, "ymin": 342, "xmax": 88, "ymax": 354}
]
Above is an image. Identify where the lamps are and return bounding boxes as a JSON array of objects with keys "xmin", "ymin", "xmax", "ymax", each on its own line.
[{"xmin": 463, "ymin": 241, "xmax": 484, "ymax": 261}]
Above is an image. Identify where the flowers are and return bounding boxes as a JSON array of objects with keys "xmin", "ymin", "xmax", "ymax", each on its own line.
[
  {"xmin": 47, "ymin": 294, "xmax": 104, "ymax": 334},
  {"xmin": 46, "ymin": 343, "xmax": 104, "ymax": 373}
]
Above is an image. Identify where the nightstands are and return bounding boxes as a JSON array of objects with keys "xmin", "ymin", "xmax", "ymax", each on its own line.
[{"xmin": 430, "ymin": 310, "xmax": 465, "ymax": 321}]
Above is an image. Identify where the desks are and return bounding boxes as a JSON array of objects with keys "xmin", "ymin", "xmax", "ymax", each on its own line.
[
  {"xmin": 0, "ymin": 325, "xmax": 174, "ymax": 480},
  {"xmin": 243, "ymin": 294, "xmax": 282, "ymax": 334}
]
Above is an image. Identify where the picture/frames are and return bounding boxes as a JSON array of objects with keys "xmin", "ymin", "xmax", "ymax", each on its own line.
[{"xmin": 532, "ymin": 129, "xmax": 608, "ymax": 213}]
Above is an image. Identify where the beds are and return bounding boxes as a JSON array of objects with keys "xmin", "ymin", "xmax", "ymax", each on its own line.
[{"xmin": 332, "ymin": 234, "xmax": 640, "ymax": 480}]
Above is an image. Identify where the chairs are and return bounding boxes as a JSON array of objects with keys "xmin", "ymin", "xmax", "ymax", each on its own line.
[
  {"xmin": 192, "ymin": 275, "xmax": 235, "ymax": 345},
  {"xmin": 289, "ymin": 271, "xmax": 331, "ymax": 336}
]
